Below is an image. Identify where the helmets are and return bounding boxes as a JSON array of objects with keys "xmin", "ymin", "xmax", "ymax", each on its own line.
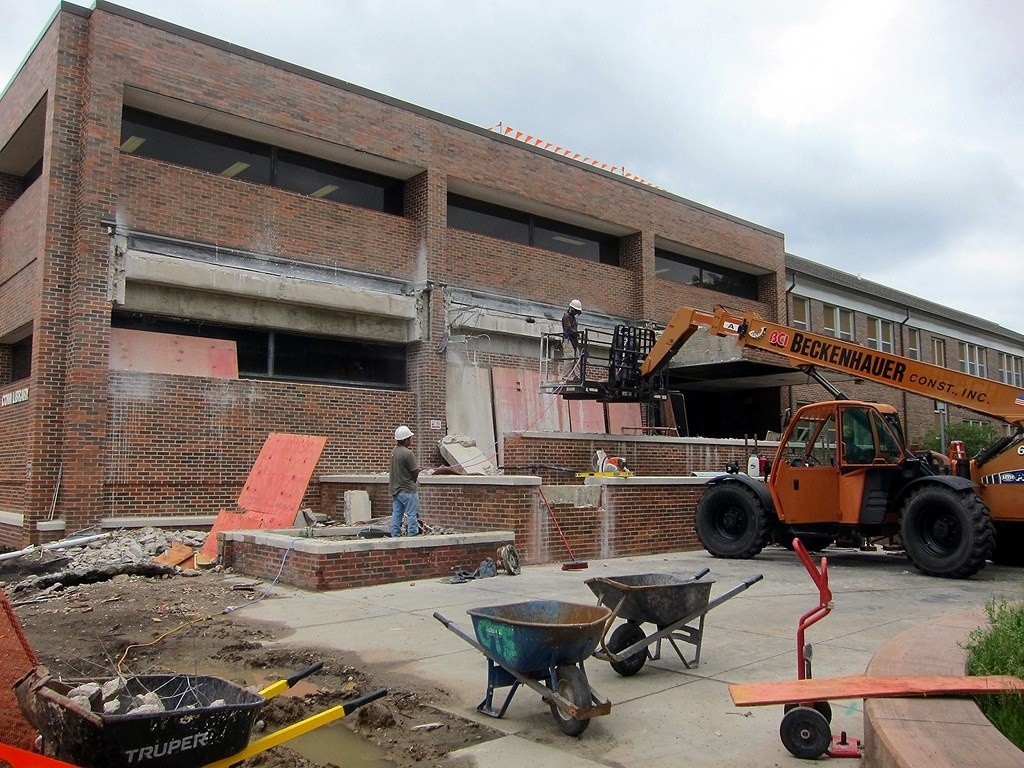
[
  {"xmin": 394, "ymin": 426, "xmax": 414, "ymax": 440},
  {"xmin": 568, "ymin": 299, "xmax": 582, "ymax": 311}
]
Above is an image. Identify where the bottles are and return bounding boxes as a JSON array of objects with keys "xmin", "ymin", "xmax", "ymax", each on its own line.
[{"xmin": 747, "ymin": 452, "xmax": 768, "ymax": 478}]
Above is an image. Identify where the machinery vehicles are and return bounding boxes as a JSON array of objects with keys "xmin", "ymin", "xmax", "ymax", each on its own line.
[{"xmin": 537, "ymin": 304, "xmax": 1024, "ymax": 579}]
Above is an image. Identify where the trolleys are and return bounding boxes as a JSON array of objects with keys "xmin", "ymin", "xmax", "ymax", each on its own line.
[
  {"xmin": 433, "ymin": 600, "xmax": 611, "ymax": 737},
  {"xmin": 11, "ymin": 663, "xmax": 388, "ymax": 768},
  {"xmin": 583, "ymin": 567, "xmax": 763, "ymax": 677},
  {"xmin": 780, "ymin": 537, "xmax": 861, "ymax": 759}
]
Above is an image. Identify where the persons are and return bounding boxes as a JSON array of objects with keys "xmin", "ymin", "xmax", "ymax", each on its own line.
[
  {"xmin": 389, "ymin": 425, "xmax": 420, "ymax": 537},
  {"xmin": 561, "ymin": 300, "xmax": 582, "ymax": 380},
  {"xmin": 844, "ymin": 426, "xmax": 867, "ymax": 464},
  {"xmin": 621, "ymin": 333, "xmax": 640, "ymax": 388}
]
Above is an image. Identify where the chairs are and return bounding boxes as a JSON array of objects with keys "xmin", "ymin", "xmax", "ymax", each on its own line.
[{"xmin": 841, "ymin": 429, "xmax": 866, "ymax": 465}]
